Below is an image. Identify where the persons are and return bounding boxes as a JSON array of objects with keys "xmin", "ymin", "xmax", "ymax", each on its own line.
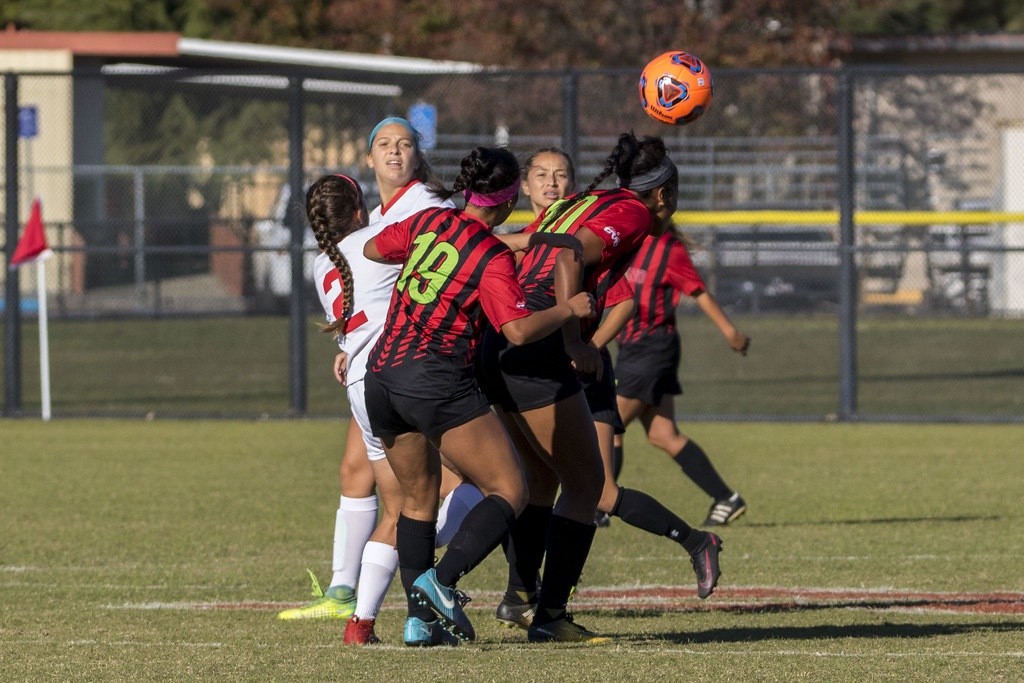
[
  {"xmin": 279, "ymin": 117, "xmax": 458, "ymax": 619},
  {"xmin": 483, "ymin": 130, "xmax": 679, "ymax": 645},
  {"xmin": 594, "ymin": 216, "xmax": 750, "ymax": 528},
  {"xmin": 501, "ymin": 149, "xmax": 723, "ymax": 599},
  {"xmin": 363, "ymin": 146, "xmax": 596, "ymax": 646},
  {"xmin": 306, "ymin": 173, "xmax": 484, "ymax": 646}
]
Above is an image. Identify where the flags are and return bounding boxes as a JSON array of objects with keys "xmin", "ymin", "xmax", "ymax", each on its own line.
[{"xmin": 11, "ymin": 201, "xmax": 49, "ymax": 266}]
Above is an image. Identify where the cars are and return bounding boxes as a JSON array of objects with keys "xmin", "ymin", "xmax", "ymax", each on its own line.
[{"xmin": 247, "ymin": 171, "xmax": 382, "ymax": 303}]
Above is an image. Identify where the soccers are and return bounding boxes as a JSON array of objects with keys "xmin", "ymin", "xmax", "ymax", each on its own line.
[{"xmin": 636, "ymin": 49, "xmax": 716, "ymax": 126}]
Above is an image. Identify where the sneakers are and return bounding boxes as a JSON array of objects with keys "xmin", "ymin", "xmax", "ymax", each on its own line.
[
  {"xmin": 409, "ymin": 565, "xmax": 480, "ymax": 646},
  {"xmin": 496, "ymin": 584, "xmax": 542, "ymax": 624},
  {"xmin": 344, "ymin": 618, "xmax": 380, "ymax": 645},
  {"xmin": 403, "ymin": 620, "xmax": 456, "ymax": 647},
  {"xmin": 275, "ymin": 570, "xmax": 355, "ymax": 622},
  {"xmin": 702, "ymin": 491, "xmax": 751, "ymax": 526},
  {"xmin": 690, "ymin": 530, "xmax": 723, "ymax": 599},
  {"xmin": 530, "ymin": 613, "xmax": 597, "ymax": 645}
]
